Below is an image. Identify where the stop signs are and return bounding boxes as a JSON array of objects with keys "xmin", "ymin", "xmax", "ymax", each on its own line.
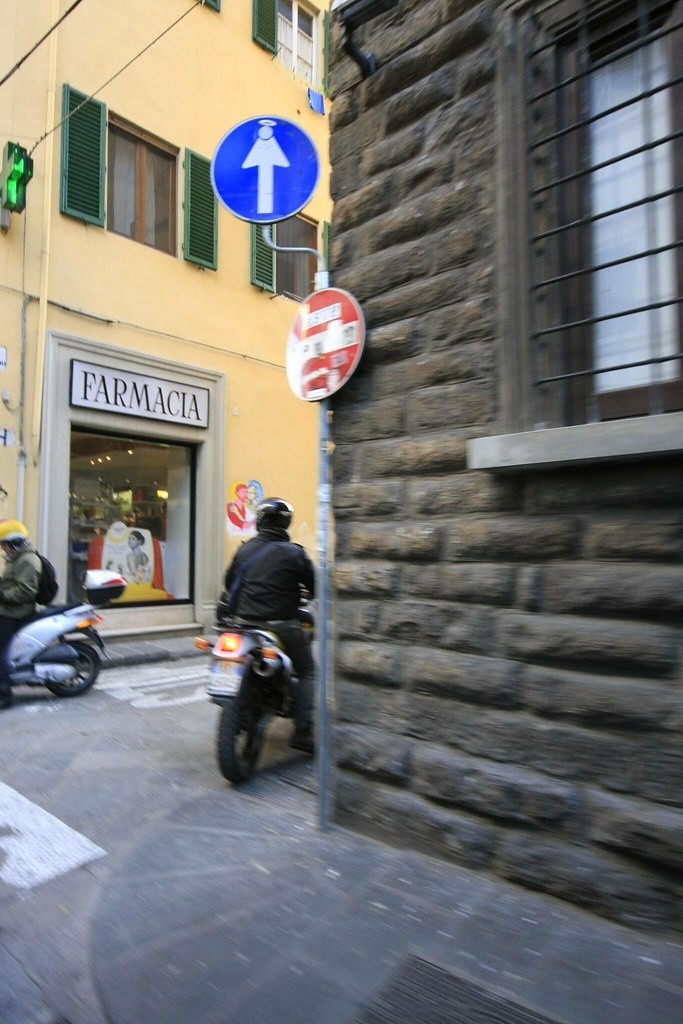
[{"xmin": 285, "ymin": 287, "xmax": 364, "ymax": 401}]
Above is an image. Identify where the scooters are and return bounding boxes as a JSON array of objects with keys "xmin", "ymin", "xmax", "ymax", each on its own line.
[{"xmin": 5, "ymin": 570, "xmax": 129, "ymax": 699}]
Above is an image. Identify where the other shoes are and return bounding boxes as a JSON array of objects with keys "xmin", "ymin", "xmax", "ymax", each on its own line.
[
  {"xmin": 0, "ymin": 696, "xmax": 12, "ymax": 708},
  {"xmin": 287, "ymin": 733, "xmax": 314, "ymax": 754}
]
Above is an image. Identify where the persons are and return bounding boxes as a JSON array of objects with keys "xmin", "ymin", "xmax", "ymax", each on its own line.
[
  {"xmin": 0, "ymin": 519, "xmax": 43, "ymax": 711},
  {"xmin": 223, "ymin": 496, "xmax": 315, "ymax": 754},
  {"xmin": 124, "ymin": 530, "xmax": 149, "ymax": 584}
]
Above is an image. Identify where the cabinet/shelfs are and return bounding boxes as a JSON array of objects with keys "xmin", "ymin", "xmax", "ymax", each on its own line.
[{"xmin": 69, "ymin": 498, "xmax": 119, "ymax": 561}]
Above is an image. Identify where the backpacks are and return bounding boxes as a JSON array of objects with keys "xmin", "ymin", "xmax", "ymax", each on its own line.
[{"xmin": 13, "ymin": 550, "xmax": 58, "ymax": 605}]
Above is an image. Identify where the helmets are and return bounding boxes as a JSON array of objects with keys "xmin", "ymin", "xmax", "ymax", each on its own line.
[
  {"xmin": 255, "ymin": 497, "xmax": 293, "ymax": 529},
  {"xmin": 0, "ymin": 520, "xmax": 30, "ymax": 542}
]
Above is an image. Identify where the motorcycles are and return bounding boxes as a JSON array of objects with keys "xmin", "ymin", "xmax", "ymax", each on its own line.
[{"xmin": 194, "ymin": 589, "xmax": 316, "ymax": 785}]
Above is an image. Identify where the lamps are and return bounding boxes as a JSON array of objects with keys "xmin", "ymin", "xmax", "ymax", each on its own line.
[{"xmin": 336, "ymin": 0, "xmax": 397, "ymax": 80}]
